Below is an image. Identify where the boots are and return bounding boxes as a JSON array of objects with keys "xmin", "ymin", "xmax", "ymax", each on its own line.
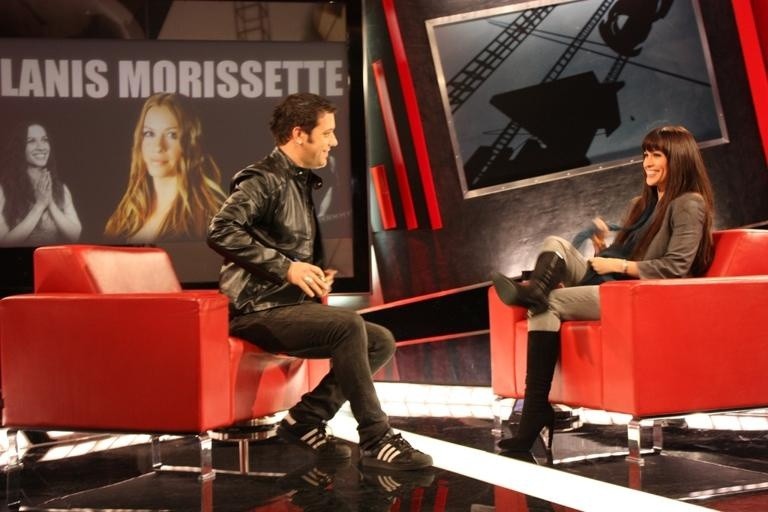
[
  {"xmin": 490, "ymin": 252, "xmax": 566, "ymax": 317},
  {"xmin": 497, "ymin": 331, "xmax": 561, "ymax": 451}
]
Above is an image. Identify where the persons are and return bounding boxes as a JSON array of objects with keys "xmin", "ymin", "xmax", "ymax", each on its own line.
[
  {"xmin": 204, "ymin": 92, "xmax": 433, "ymax": 470},
  {"xmin": 492, "ymin": 123, "xmax": 718, "ymax": 455},
  {"xmin": 102, "ymin": 91, "xmax": 228, "ymax": 239},
  {"xmin": 0, "ymin": 119, "xmax": 81, "ymax": 249},
  {"xmin": 280, "ymin": 471, "xmax": 435, "ymax": 510}
]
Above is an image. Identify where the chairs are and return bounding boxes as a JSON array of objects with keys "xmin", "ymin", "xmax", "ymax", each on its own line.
[
  {"xmin": 488, "ymin": 228, "xmax": 767, "ymax": 466},
  {"xmin": 0, "ymin": 245, "xmax": 330, "ymax": 481}
]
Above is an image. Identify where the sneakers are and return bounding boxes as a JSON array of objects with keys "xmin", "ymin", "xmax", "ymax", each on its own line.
[
  {"xmin": 297, "ymin": 461, "xmax": 337, "ymax": 492},
  {"xmin": 358, "ymin": 467, "xmax": 434, "ymax": 498},
  {"xmin": 359, "ymin": 432, "xmax": 433, "ymax": 470},
  {"xmin": 275, "ymin": 412, "xmax": 352, "ymax": 461}
]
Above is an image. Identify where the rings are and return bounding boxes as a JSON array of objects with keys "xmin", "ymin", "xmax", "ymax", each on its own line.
[{"xmin": 301, "ymin": 275, "xmax": 314, "ymax": 285}]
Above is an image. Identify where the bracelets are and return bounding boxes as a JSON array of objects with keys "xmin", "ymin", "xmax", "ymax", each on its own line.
[{"xmin": 623, "ymin": 258, "xmax": 628, "ymax": 276}]
